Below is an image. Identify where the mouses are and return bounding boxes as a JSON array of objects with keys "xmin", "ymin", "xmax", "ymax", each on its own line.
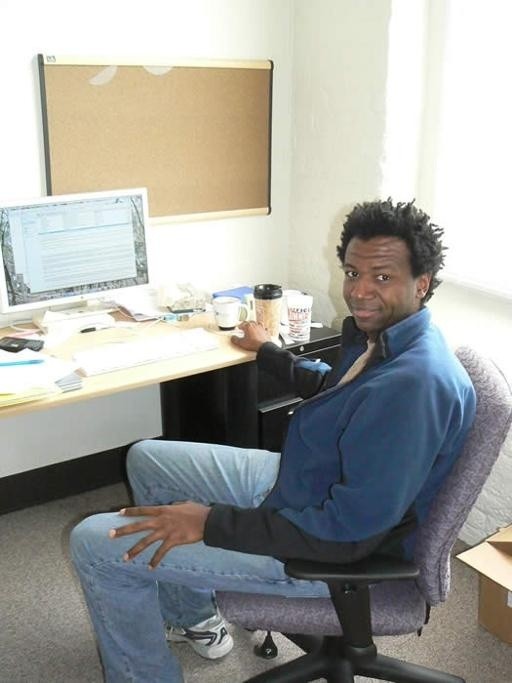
[{"xmin": 228, "ymin": 328, "xmax": 245, "ymax": 340}]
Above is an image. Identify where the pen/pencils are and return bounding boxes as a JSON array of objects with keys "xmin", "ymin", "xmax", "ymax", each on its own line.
[
  {"xmin": 1, "ymin": 361, "xmax": 43, "ymax": 365},
  {"xmin": 81, "ymin": 326, "xmax": 115, "ymax": 333}
]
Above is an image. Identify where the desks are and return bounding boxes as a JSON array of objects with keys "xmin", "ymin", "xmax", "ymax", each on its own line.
[{"xmin": 0, "ymin": 308, "xmax": 342, "ymax": 454}]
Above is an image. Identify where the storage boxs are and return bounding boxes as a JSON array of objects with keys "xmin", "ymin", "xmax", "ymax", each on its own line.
[{"xmin": 452, "ymin": 524, "xmax": 512, "ymax": 646}]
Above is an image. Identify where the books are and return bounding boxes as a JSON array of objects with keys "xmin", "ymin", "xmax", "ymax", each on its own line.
[{"xmin": 0, "ymin": 370, "xmax": 82, "ymax": 409}]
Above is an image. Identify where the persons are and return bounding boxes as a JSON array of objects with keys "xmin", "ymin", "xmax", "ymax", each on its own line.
[{"xmin": 67, "ymin": 196, "xmax": 477, "ymax": 683}]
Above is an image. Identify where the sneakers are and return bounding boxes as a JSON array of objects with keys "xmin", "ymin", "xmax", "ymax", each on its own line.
[{"xmin": 163, "ymin": 607, "xmax": 234, "ymax": 660}]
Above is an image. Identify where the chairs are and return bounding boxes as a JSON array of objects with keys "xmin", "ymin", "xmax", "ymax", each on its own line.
[{"xmin": 216, "ymin": 347, "xmax": 512, "ymax": 683}]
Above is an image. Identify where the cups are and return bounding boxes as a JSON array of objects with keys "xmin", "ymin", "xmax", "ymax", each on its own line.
[
  {"xmin": 286, "ymin": 294, "xmax": 314, "ymax": 344},
  {"xmin": 253, "ymin": 283, "xmax": 283, "ymax": 337},
  {"xmin": 212, "ymin": 297, "xmax": 248, "ymax": 331}
]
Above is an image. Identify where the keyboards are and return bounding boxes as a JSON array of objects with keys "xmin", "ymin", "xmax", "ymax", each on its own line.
[{"xmin": 73, "ymin": 327, "xmax": 220, "ymax": 375}]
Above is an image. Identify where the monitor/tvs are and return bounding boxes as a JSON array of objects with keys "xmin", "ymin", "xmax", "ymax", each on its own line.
[{"xmin": 1, "ymin": 187, "xmax": 151, "ymax": 325}]
